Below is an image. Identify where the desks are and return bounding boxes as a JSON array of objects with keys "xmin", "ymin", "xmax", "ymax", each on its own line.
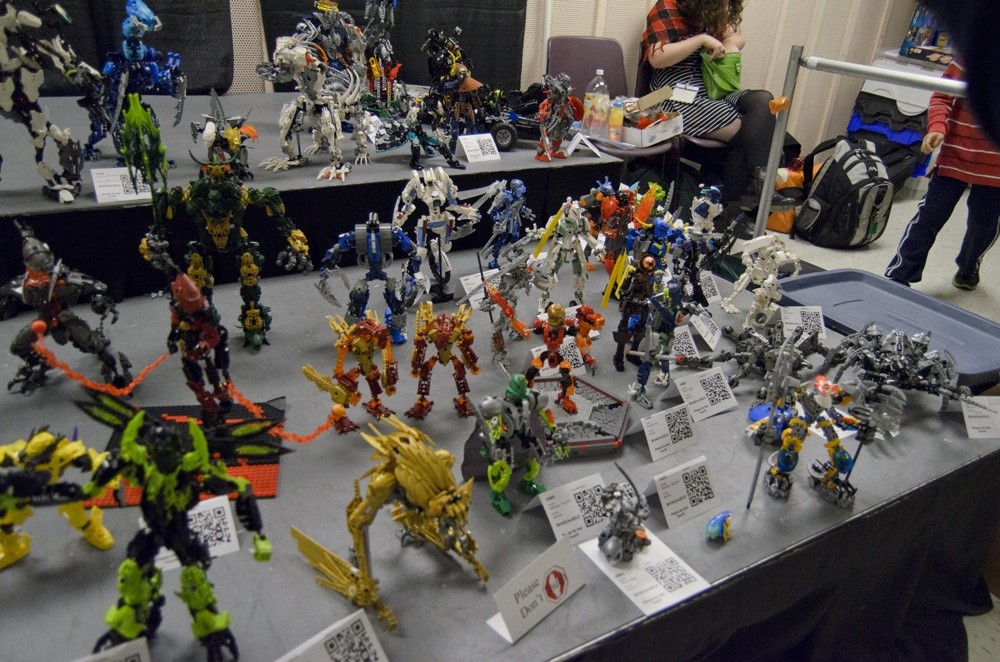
[{"xmin": 0, "ymin": 235, "xmax": 1000, "ymax": 661}]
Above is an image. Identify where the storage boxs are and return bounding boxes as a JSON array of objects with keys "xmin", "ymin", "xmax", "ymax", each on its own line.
[
  {"xmin": 621, "ymin": 112, "xmax": 683, "ymax": 147},
  {"xmin": 900, "ymin": 2, "xmax": 955, "ymax": 67}
]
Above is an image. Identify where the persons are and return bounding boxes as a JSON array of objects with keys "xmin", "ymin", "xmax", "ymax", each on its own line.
[
  {"xmin": 885, "ymin": 56, "xmax": 1000, "ymax": 289},
  {"xmin": 641, "ymin": 0, "xmax": 795, "ymax": 240}
]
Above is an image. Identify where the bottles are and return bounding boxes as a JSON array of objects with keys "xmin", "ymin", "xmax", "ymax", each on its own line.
[
  {"xmin": 581, "ymin": 69, "xmax": 610, "ymax": 136},
  {"xmin": 608, "ymin": 103, "xmax": 623, "ymax": 142}
]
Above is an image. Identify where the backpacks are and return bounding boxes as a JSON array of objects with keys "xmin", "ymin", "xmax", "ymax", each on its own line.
[{"xmin": 788, "ymin": 135, "xmax": 895, "ymax": 249}]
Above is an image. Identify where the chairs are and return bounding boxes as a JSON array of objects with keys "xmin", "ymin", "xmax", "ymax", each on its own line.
[
  {"xmin": 634, "ymin": 38, "xmax": 735, "ymax": 184},
  {"xmin": 547, "ymin": 36, "xmax": 672, "ymax": 194}
]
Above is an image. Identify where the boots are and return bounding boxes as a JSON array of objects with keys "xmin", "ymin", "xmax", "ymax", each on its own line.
[
  {"xmin": 708, "ymin": 197, "xmax": 766, "ymax": 241},
  {"xmin": 754, "ymin": 164, "xmax": 795, "ymax": 205}
]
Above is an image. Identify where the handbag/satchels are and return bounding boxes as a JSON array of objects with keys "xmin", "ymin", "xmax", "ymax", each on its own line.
[{"xmin": 700, "ymin": 46, "xmax": 742, "ymax": 100}]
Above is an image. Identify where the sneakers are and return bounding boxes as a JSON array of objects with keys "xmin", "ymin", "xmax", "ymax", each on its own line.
[{"xmin": 953, "ymin": 263, "xmax": 982, "ymax": 290}]
